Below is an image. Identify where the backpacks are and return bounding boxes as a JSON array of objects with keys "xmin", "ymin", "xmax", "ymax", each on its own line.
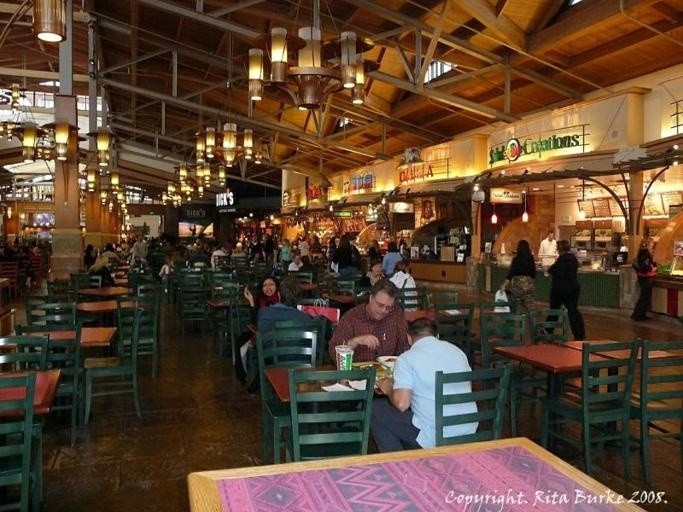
[{"xmin": 494, "ymin": 290, "xmax": 511, "ymax": 313}]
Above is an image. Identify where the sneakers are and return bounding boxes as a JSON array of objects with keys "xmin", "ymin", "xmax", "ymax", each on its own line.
[{"xmin": 630, "ymin": 313, "xmax": 653, "ymax": 322}]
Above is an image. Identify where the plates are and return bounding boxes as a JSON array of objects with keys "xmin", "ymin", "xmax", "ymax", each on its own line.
[
  {"xmin": 377, "ymin": 356, "xmax": 398, "ymax": 367},
  {"xmin": 359, "ymin": 363, "xmax": 391, "ymax": 379}
]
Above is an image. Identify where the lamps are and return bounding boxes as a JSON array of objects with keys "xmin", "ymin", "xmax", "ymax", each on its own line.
[
  {"xmin": 577, "ymin": 179, "xmax": 587, "ymax": 221},
  {"xmin": 521, "ymin": 181, "xmax": 529, "ymax": 222},
  {"xmin": 193, "ymin": 78, "xmax": 264, "ymax": 168},
  {"xmin": 159, "ymin": 146, "xmax": 229, "ymax": 210},
  {"xmin": 489, "ymin": 180, "xmax": 498, "ymax": 224},
  {"xmin": 238, "ymin": 0, "xmax": 368, "ymax": 113},
  {"xmin": 1, "ymin": 85, "xmax": 135, "ymax": 240},
  {"xmin": 30, "ymin": 1, "xmax": 69, "ymax": 44}
]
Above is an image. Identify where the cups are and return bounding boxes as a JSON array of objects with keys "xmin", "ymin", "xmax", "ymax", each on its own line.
[{"xmin": 333, "ymin": 345, "xmax": 354, "ymax": 371}]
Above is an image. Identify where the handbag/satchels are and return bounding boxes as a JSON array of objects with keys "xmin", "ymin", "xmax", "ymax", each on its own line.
[
  {"xmin": 296, "ymin": 297, "xmax": 342, "ymax": 326},
  {"xmin": 630, "ymin": 257, "xmax": 649, "ymax": 275},
  {"xmin": 351, "ymin": 251, "xmax": 361, "ymax": 264}
]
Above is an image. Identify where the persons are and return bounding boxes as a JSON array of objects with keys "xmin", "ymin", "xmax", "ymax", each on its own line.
[
  {"xmin": 544, "ymin": 240, "xmax": 588, "ymax": 340},
  {"xmin": 537, "ymin": 231, "xmax": 560, "ymax": 270},
  {"xmin": 235, "ymin": 275, "xmax": 288, "ymax": 399},
  {"xmin": 328, "ymin": 278, "xmax": 410, "ymax": 365},
  {"xmin": 501, "ymin": 239, "xmax": 537, "ymax": 323},
  {"xmin": 0, "ymin": 230, "xmax": 432, "ymax": 311},
  {"xmin": 370, "ymin": 316, "xmax": 480, "ymax": 454},
  {"xmin": 629, "ymin": 237, "xmax": 658, "ymax": 322}
]
